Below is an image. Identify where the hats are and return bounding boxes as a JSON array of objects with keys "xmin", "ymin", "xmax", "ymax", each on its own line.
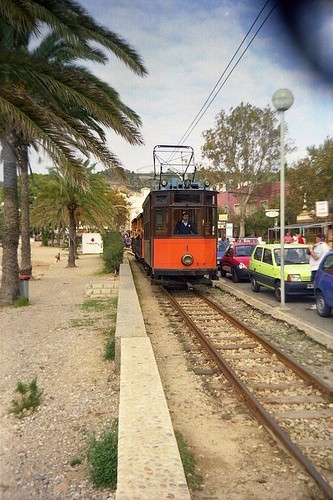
[{"xmin": 318, "ymin": 233, "xmax": 327, "ymax": 240}]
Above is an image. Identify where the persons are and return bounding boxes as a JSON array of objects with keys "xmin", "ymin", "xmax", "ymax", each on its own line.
[
  {"xmin": 284, "ymin": 230, "xmax": 307, "ymax": 243},
  {"xmin": 307, "ymin": 233, "xmax": 330, "ymax": 309},
  {"xmin": 174, "ymin": 210, "xmax": 199, "ymax": 234}
]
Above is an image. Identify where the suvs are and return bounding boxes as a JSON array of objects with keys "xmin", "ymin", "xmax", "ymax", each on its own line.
[{"xmin": 248, "ymin": 244, "xmax": 315, "ymax": 302}]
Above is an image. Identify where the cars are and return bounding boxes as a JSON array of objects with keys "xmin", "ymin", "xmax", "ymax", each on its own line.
[
  {"xmin": 314, "ymin": 251, "xmax": 333, "ymax": 317},
  {"xmin": 218, "ymin": 241, "xmax": 269, "ymax": 283}
]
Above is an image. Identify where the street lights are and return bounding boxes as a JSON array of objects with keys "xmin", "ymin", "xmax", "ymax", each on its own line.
[{"xmin": 272, "ymin": 89, "xmax": 294, "ymax": 309}]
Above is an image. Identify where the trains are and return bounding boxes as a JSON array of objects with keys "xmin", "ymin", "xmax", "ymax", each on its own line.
[{"xmin": 76, "ymin": 178, "xmax": 220, "ymax": 291}]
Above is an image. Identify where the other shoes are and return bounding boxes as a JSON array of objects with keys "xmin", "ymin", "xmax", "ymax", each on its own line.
[{"xmin": 311, "ymin": 304, "xmax": 317, "ymax": 309}]
